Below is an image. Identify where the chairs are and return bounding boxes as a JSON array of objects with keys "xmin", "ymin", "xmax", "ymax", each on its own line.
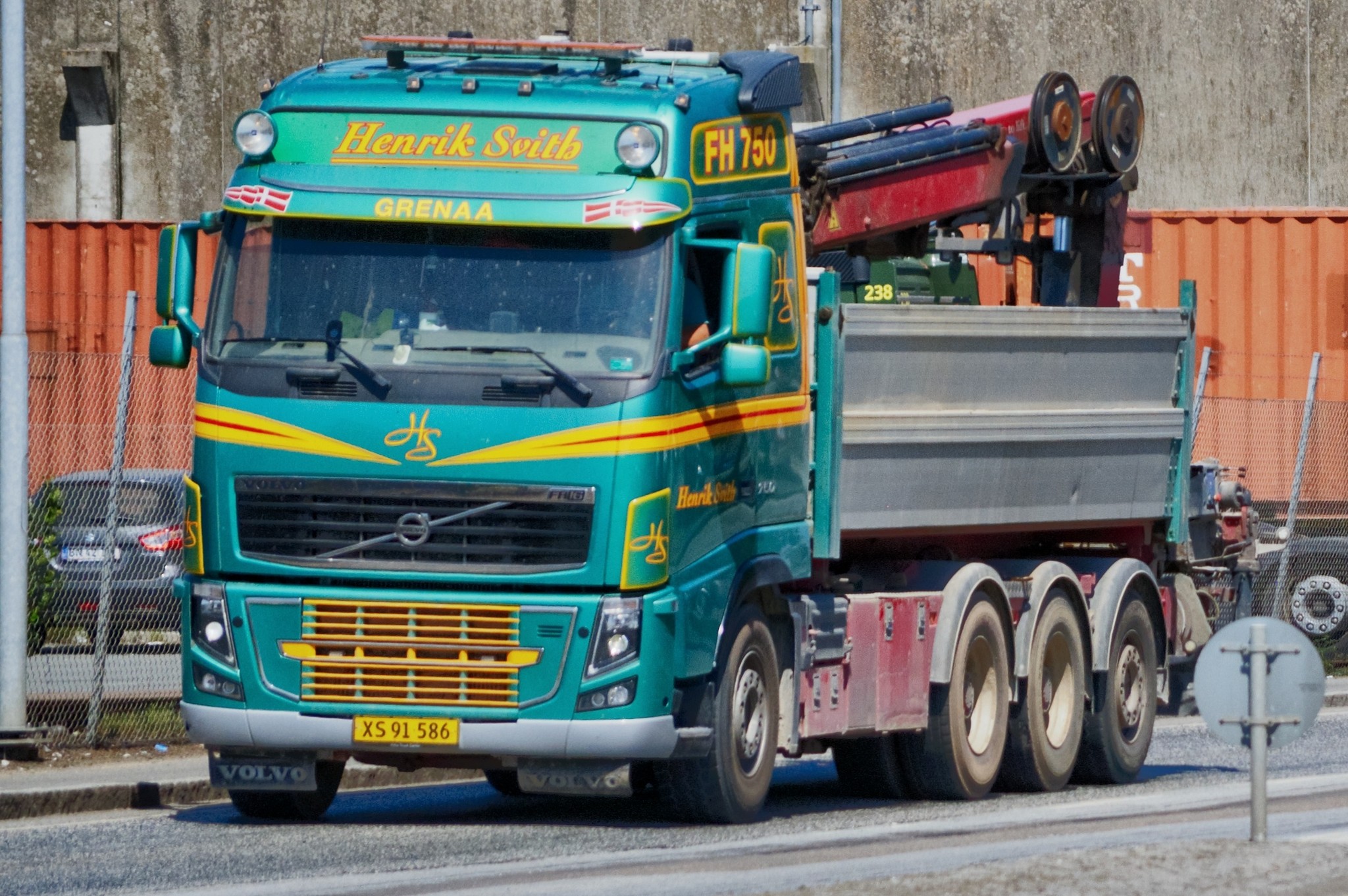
[{"xmin": 87, "ymin": 490, "xmax": 109, "ymax": 516}]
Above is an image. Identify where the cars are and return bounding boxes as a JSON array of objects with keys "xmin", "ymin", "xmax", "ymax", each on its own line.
[{"xmin": 28, "ymin": 468, "xmax": 194, "ymax": 657}]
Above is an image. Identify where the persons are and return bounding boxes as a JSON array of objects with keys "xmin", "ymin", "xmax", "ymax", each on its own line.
[{"xmin": 607, "ymin": 228, "xmax": 711, "ymax": 350}]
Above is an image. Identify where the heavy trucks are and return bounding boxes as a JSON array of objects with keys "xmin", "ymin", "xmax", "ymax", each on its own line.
[{"xmin": 148, "ymin": 27, "xmax": 1186, "ymax": 827}]
